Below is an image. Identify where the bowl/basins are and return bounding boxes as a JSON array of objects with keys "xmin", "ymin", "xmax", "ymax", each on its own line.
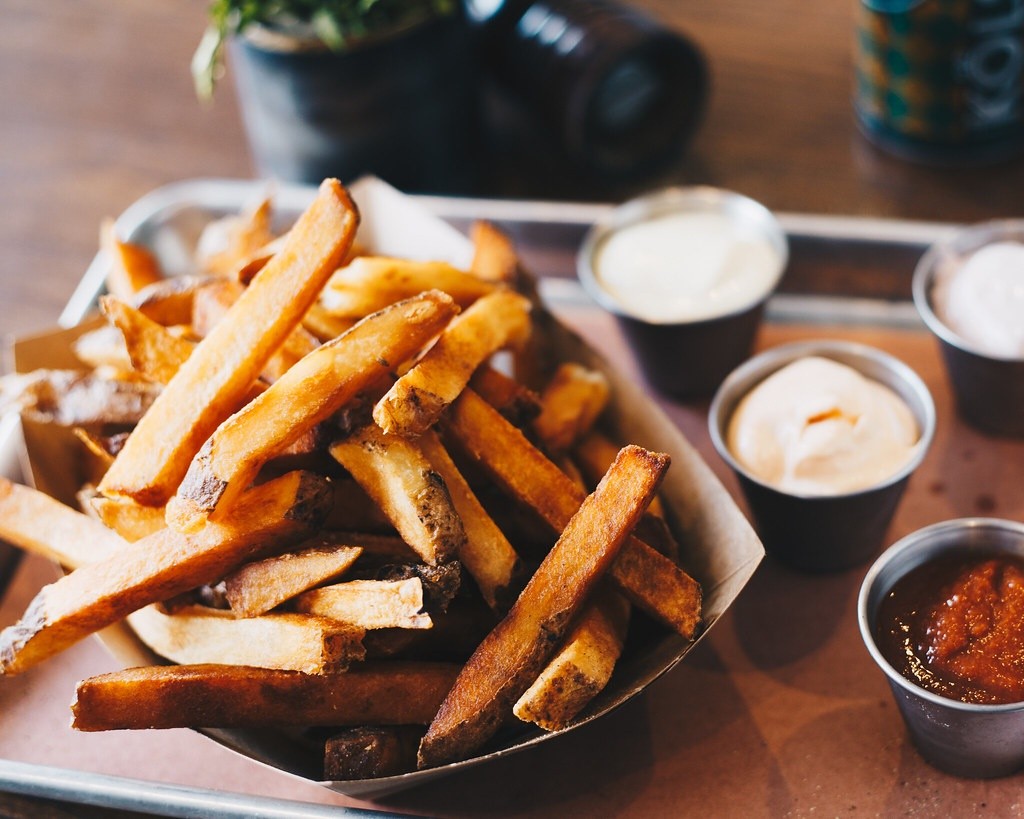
[
  {"xmin": 910, "ymin": 216, "xmax": 1024, "ymax": 439},
  {"xmin": 858, "ymin": 517, "xmax": 1024, "ymax": 780},
  {"xmin": 575, "ymin": 184, "xmax": 789, "ymax": 405},
  {"xmin": 707, "ymin": 338, "xmax": 937, "ymax": 575}
]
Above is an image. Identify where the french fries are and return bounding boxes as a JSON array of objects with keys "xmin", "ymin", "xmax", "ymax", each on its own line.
[{"xmin": 0, "ymin": 174, "xmax": 706, "ymax": 780}]
